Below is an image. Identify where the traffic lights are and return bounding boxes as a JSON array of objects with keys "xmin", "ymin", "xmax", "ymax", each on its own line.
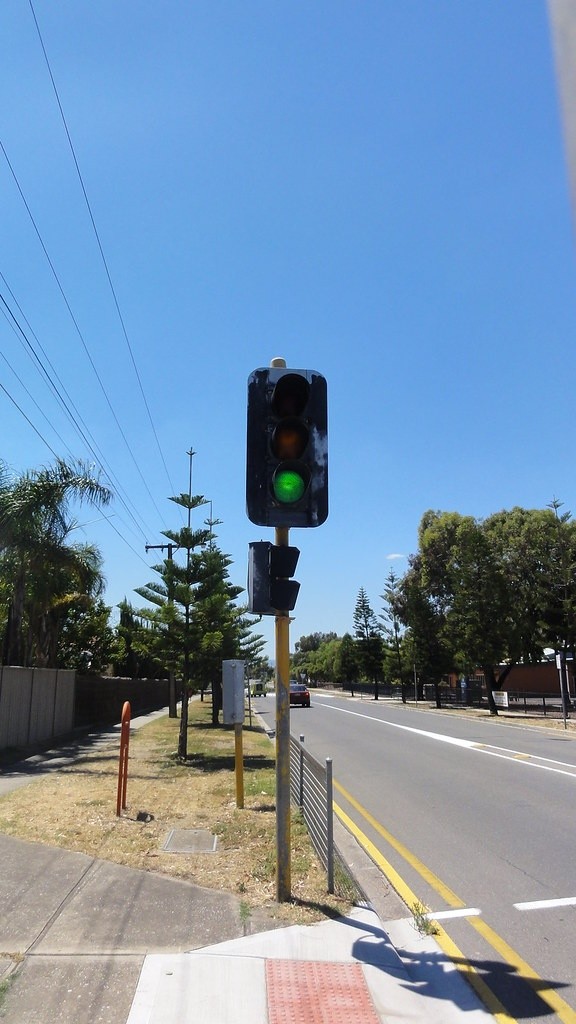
[
  {"xmin": 246, "ymin": 368, "xmax": 331, "ymax": 529},
  {"xmin": 244, "ymin": 541, "xmax": 302, "ymax": 616}
]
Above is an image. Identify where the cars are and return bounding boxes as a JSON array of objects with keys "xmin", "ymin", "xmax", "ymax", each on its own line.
[
  {"xmin": 244, "ymin": 681, "xmax": 267, "ymax": 698},
  {"xmin": 289, "ymin": 683, "xmax": 311, "ymax": 708}
]
